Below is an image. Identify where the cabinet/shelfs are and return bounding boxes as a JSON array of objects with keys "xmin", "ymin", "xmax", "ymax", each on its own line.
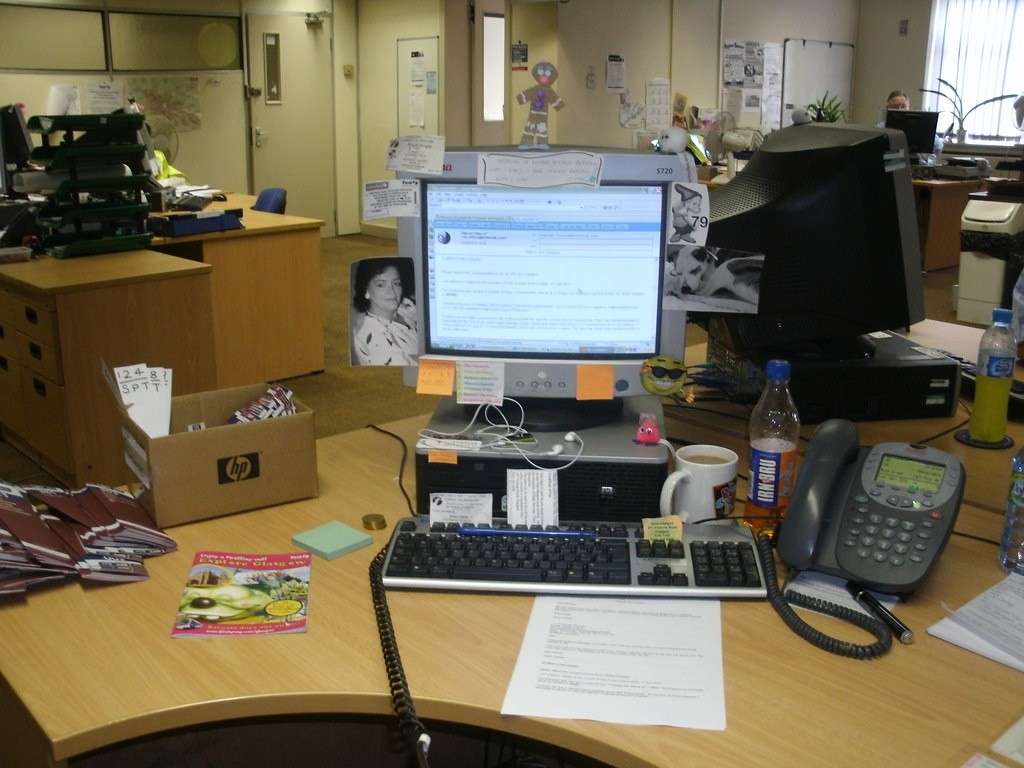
[{"xmin": 0, "ymin": 250, "xmax": 213, "ymax": 495}]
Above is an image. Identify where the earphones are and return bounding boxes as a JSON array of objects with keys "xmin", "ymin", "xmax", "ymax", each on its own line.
[
  {"xmin": 564, "ymin": 431, "xmax": 582, "ymax": 443},
  {"xmin": 547, "ymin": 444, "xmax": 563, "ymax": 456}
]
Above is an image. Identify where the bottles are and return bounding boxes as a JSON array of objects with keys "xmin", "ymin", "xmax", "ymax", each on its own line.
[
  {"xmin": 998, "ymin": 444, "xmax": 1024, "ymax": 577},
  {"xmin": 1010, "ymin": 267, "xmax": 1024, "ymax": 362},
  {"xmin": 968, "ymin": 308, "xmax": 1018, "ymax": 444},
  {"xmin": 744, "ymin": 359, "xmax": 800, "ymax": 536},
  {"xmin": 927, "ymin": 152, "xmax": 937, "ymax": 166}
]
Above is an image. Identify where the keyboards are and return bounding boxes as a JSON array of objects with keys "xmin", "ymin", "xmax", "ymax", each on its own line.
[
  {"xmin": 377, "ymin": 515, "xmax": 768, "ymax": 600},
  {"xmin": 172, "ymin": 195, "xmax": 213, "ymax": 211},
  {"xmin": 935, "ymin": 349, "xmax": 1024, "ymax": 414}
]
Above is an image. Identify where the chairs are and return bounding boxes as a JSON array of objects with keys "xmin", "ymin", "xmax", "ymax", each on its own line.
[{"xmin": 254, "ymin": 187, "xmax": 287, "ymax": 214}]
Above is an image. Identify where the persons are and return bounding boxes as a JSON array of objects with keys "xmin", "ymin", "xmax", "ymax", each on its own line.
[
  {"xmin": 875, "ymin": 90, "xmax": 944, "ymax": 165},
  {"xmin": 351, "ymin": 256, "xmax": 418, "ymax": 366}
]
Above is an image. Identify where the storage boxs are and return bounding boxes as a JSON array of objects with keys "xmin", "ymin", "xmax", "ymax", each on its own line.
[{"xmin": 116, "ymin": 382, "xmax": 319, "ymax": 529}]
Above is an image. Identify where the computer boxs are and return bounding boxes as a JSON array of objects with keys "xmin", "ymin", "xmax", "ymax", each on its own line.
[
  {"xmin": 415, "ymin": 394, "xmax": 669, "ymax": 521},
  {"xmin": 705, "ymin": 330, "xmax": 963, "ymax": 425}
]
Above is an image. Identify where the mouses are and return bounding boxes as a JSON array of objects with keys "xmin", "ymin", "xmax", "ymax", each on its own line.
[{"xmin": 212, "ymin": 194, "xmax": 227, "ymax": 201}]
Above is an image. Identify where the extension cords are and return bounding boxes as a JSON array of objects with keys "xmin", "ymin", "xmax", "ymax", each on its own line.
[{"xmin": 416, "ymin": 437, "xmax": 482, "ymax": 459}]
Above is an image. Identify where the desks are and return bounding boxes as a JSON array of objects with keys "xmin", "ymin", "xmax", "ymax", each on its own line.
[
  {"xmin": 147, "ymin": 194, "xmax": 326, "ymax": 390},
  {"xmin": 1, "ymin": 341, "xmax": 1024, "ymax": 767},
  {"xmin": 911, "ymin": 178, "xmax": 982, "ymax": 277}
]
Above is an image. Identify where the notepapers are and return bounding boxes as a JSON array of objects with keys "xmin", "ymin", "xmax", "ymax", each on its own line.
[{"xmin": 292, "ymin": 519, "xmax": 374, "ymax": 560}]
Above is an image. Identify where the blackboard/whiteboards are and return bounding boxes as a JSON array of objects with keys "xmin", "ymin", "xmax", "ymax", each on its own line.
[{"xmin": 779, "ymin": 38, "xmax": 854, "ymax": 129}]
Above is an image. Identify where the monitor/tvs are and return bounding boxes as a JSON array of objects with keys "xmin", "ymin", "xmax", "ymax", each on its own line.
[
  {"xmin": 398, "ymin": 146, "xmax": 693, "ymax": 432},
  {"xmin": 689, "ymin": 121, "xmax": 928, "ymax": 357},
  {"xmin": 0, "ymin": 103, "xmax": 34, "ymax": 196},
  {"xmin": 885, "ymin": 109, "xmax": 939, "ymax": 158}
]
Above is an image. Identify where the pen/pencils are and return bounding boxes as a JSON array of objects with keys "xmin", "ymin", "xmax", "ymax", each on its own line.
[{"xmin": 846, "ymin": 581, "xmax": 914, "ymax": 645}]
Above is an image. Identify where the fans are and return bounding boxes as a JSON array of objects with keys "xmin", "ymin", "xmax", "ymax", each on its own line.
[{"xmin": 703, "ymin": 112, "xmax": 750, "ymax": 185}]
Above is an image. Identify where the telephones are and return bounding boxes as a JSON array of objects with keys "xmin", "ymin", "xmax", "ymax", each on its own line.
[{"xmin": 776, "ymin": 418, "xmax": 968, "ymax": 594}]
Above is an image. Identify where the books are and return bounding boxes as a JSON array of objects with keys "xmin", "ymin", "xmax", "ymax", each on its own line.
[{"xmin": 0, "ymin": 480, "xmax": 312, "ymax": 639}]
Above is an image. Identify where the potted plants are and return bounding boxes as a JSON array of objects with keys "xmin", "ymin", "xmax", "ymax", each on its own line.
[{"xmin": 919, "ymin": 77, "xmax": 1018, "ymax": 141}]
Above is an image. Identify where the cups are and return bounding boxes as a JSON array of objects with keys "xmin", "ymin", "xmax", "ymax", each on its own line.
[{"xmin": 660, "ymin": 444, "xmax": 738, "ymax": 526}]
